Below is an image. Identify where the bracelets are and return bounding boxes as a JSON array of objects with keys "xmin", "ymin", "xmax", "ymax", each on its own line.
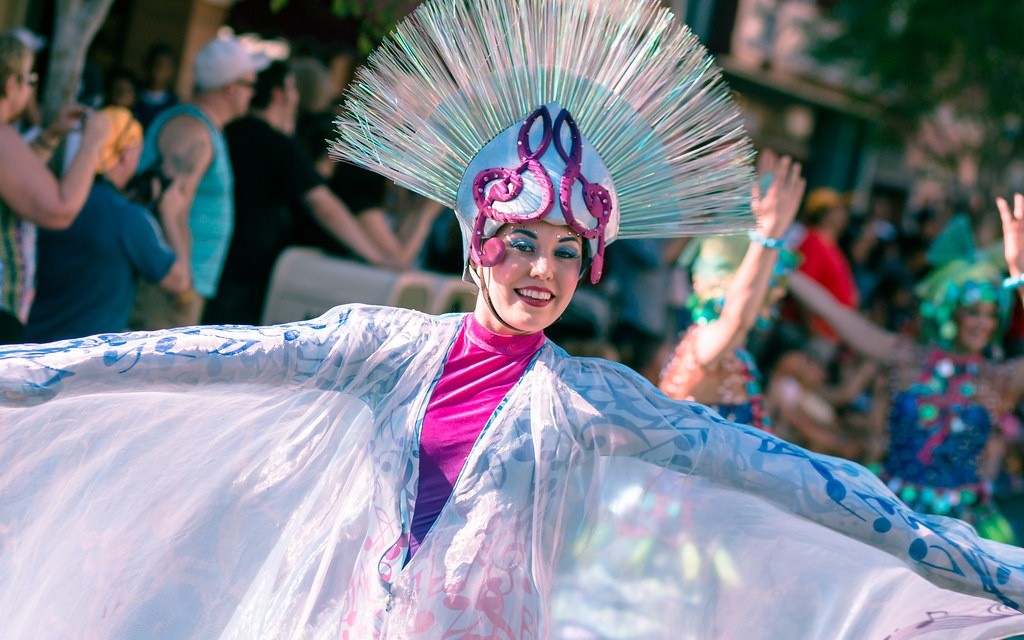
[
  {"xmin": 35, "ymin": 136, "xmax": 56, "ymax": 151},
  {"xmin": 747, "ymin": 229, "xmax": 785, "ymax": 249},
  {"xmin": 1002, "ymin": 273, "xmax": 1024, "ymax": 290}
]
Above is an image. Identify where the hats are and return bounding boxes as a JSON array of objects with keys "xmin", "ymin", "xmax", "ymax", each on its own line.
[
  {"xmin": 94, "ymin": 106, "xmax": 142, "ymax": 177},
  {"xmin": 806, "ymin": 188, "xmax": 853, "ymax": 215},
  {"xmin": 194, "ymin": 39, "xmax": 273, "ymax": 93}
]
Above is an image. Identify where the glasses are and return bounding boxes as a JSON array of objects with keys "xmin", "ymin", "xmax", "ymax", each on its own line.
[
  {"xmin": 233, "ymin": 75, "xmax": 261, "ymax": 90},
  {"xmin": 20, "ymin": 69, "xmax": 40, "ymax": 84}
]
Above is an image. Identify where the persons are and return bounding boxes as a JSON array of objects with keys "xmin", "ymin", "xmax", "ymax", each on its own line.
[
  {"xmin": 0, "ymin": 9, "xmax": 1024, "ymax": 569},
  {"xmin": 1, "ymin": 164, "xmax": 1024, "ymax": 639}
]
